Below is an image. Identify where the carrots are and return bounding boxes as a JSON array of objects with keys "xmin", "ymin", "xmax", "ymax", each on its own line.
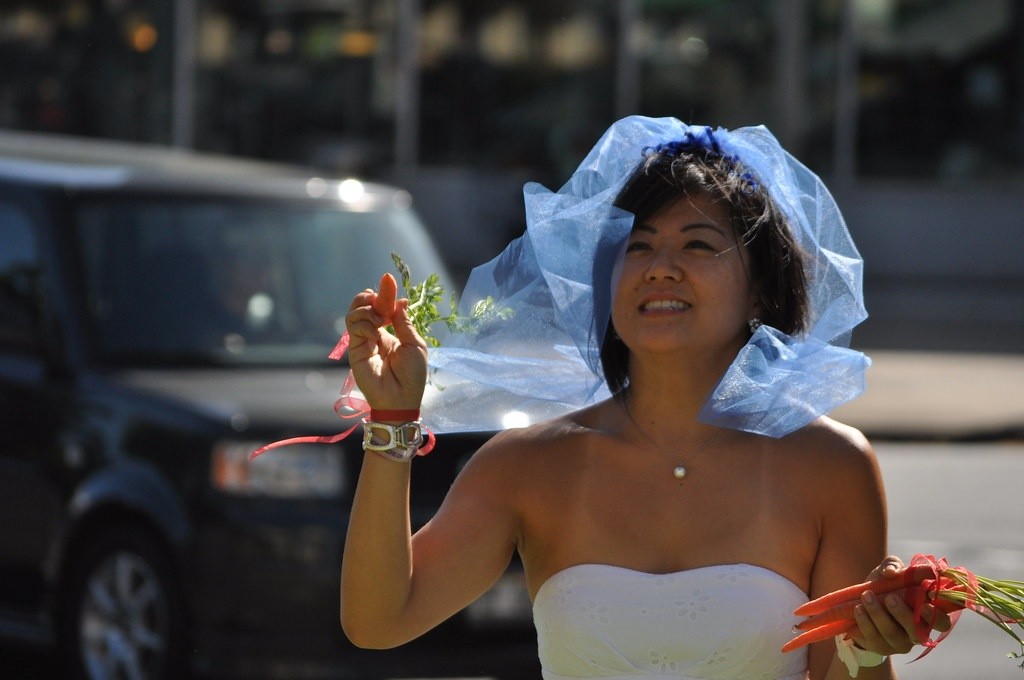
[{"xmin": 781, "ymin": 563, "xmax": 974, "ymax": 653}]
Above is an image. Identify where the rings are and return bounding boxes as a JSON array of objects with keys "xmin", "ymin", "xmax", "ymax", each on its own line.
[{"xmin": 909, "ymin": 637, "xmax": 920, "ymax": 645}]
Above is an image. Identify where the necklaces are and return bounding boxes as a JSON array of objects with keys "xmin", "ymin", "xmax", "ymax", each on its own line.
[{"xmin": 622, "ymin": 389, "xmax": 725, "ymax": 479}]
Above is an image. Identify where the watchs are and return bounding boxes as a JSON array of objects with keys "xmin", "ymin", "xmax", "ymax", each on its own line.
[{"xmin": 835, "ymin": 632, "xmax": 888, "ymax": 679}]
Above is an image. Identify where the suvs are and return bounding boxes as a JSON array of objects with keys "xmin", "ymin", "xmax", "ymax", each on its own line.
[{"xmin": 1, "ymin": 125, "xmax": 606, "ymax": 680}]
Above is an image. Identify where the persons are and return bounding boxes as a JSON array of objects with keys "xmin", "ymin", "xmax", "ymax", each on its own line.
[{"xmin": 340, "ymin": 115, "xmax": 969, "ymax": 680}]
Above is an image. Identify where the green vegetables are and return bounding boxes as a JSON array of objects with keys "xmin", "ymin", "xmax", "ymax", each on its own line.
[{"xmin": 385, "ymin": 252, "xmax": 514, "ymax": 346}]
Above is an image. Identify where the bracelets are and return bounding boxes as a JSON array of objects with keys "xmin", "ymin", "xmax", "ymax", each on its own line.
[{"xmin": 362, "ymin": 409, "xmax": 423, "ymax": 463}]
